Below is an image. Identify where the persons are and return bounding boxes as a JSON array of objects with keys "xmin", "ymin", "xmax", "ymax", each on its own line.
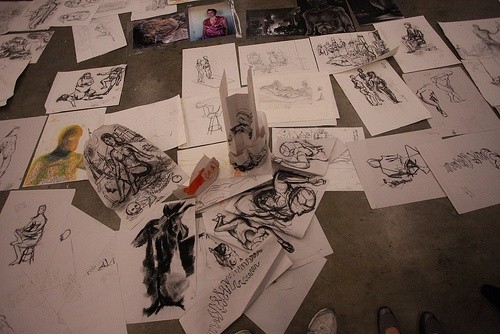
[{"xmin": 201, "ymin": 8, "xmax": 229, "ymax": 41}]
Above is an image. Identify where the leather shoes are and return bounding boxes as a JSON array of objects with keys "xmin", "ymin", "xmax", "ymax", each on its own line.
[{"xmin": 377, "ymin": 306, "xmax": 400, "ymax": 334}]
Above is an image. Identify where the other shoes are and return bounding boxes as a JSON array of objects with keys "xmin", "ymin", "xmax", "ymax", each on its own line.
[
  {"xmin": 480, "ymin": 283, "xmax": 500, "ymax": 308},
  {"xmin": 421, "ymin": 311, "xmax": 445, "ymax": 334}
]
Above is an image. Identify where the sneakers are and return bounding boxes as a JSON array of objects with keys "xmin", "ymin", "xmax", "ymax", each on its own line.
[
  {"xmin": 235, "ymin": 330, "xmax": 253, "ymax": 334},
  {"xmin": 305, "ymin": 307, "xmax": 337, "ymax": 334}
]
